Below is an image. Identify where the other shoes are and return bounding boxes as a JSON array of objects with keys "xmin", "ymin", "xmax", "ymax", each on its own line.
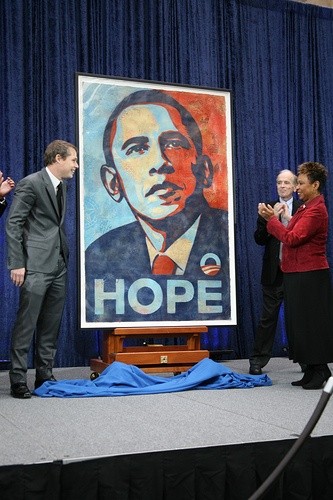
[{"xmin": 291, "ymin": 363, "xmax": 332, "ymax": 390}]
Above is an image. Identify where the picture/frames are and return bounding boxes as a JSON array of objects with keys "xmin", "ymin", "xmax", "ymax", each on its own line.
[{"xmin": 75, "ymin": 71, "xmax": 239, "ymax": 332}]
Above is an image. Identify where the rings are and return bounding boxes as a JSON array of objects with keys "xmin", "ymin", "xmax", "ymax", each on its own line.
[{"xmin": 262, "ymin": 210, "xmax": 266, "ymax": 214}]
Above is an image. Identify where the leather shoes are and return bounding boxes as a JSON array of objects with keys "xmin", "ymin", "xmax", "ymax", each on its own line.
[
  {"xmin": 249, "ymin": 365, "xmax": 262, "ymax": 375},
  {"xmin": 34, "ymin": 375, "xmax": 57, "ymax": 389},
  {"xmin": 10, "ymin": 384, "xmax": 32, "ymax": 399}
]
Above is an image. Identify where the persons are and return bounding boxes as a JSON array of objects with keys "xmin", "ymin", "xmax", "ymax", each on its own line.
[
  {"xmin": 258, "ymin": 162, "xmax": 333, "ymax": 389},
  {"xmin": 6, "ymin": 140, "xmax": 79, "ymax": 397},
  {"xmin": 0, "ymin": 170, "xmax": 15, "ymax": 218},
  {"xmin": 248, "ymin": 170, "xmax": 303, "ymax": 374}
]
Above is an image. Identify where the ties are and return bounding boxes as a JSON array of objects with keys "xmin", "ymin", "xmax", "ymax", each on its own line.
[{"xmin": 56, "ymin": 181, "xmax": 63, "ymax": 219}]
